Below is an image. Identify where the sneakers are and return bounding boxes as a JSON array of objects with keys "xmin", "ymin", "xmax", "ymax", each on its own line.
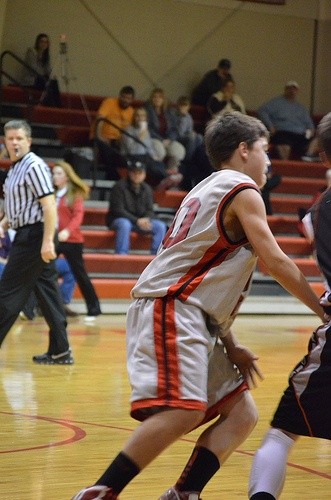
[
  {"xmin": 71, "ymin": 484, "xmax": 117, "ymax": 500},
  {"xmin": 33, "ymin": 350, "xmax": 73, "ymax": 364},
  {"xmin": 157, "ymin": 486, "xmax": 201, "ymax": 500}
]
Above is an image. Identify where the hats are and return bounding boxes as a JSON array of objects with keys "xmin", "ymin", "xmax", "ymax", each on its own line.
[
  {"xmin": 285, "ymin": 80, "xmax": 299, "ymax": 89},
  {"xmin": 129, "ymin": 161, "xmax": 146, "ymax": 171}
]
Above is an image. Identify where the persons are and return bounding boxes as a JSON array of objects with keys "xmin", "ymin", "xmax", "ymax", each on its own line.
[
  {"xmin": 71, "ymin": 110, "xmax": 331, "ymax": 500},
  {"xmin": 249, "ymin": 113, "xmax": 331, "ymax": 500},
  {"xmin": 0, "ymin": 183, "xmax": 76, "ymax": 321},
  {"xmin": 20, "ymin": 33, "xmax": 61, "ymax": 107},
  {"xmin": 105, "ymin": 161, "xmax": 166, "ymax": 254},
  {"xmin": 0, "ymin": 120, "xmax": 75, "ymax": 365},
  {"xmin": 51, "ymin": 162, "xmax": 101, "ymax": 316},
  {"xmin": 94, "ymin": 58, "xmax": 323, "ymax": 216}
]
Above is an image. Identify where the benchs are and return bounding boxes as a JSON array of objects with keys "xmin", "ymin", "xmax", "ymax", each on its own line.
[{"xmin": 0, "ymin": 84, "xmax": 331, "ymax": 281}]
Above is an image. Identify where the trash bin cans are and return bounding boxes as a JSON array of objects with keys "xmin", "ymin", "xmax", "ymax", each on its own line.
[{"xmin": 64, "ymin": 143, "xmax": 93, "ymax": 178}]
